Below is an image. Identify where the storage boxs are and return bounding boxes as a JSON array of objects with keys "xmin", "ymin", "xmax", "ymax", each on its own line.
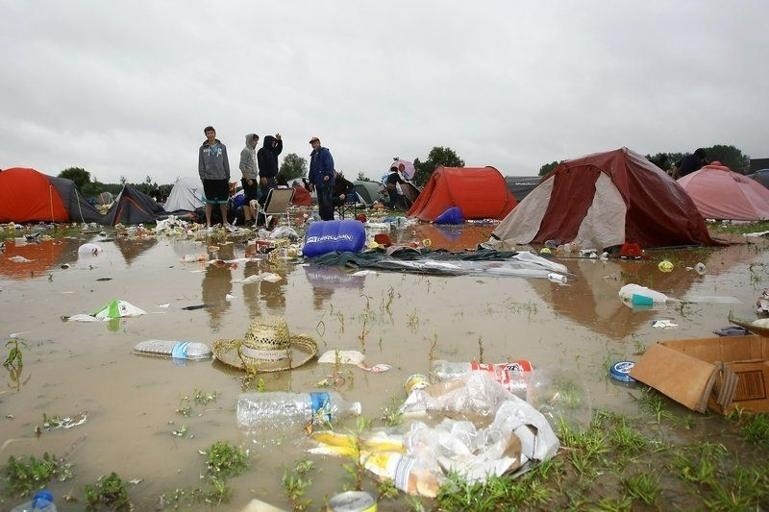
[{"xmin": 628, "ymin": 334, "xmax": 769, "ymax": 417}]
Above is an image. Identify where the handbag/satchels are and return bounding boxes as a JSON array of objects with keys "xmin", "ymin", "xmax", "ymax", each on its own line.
[{"xmin": 387, "ymin": 182, "xmax": 394, "ymax": 193}]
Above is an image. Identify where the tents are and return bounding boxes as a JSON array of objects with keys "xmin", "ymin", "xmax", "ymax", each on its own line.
[
  {"xmin": 745, "ymin": 169, "xmax": 769, "ymax": 189},
  {"xmin": 675, "ymin": 161, "xmax": 769, "ymax": 221},
  {"xmin": 0, "ymin": 168, "xmax": 102, "ymax": 223},
  {"xmin": 405, "ymin": 166, "xmax": 517, "ymax": 221},
  {"xmin": 488, "ymin": 146, "xmax": 730, "ymax": 253},
  {"xmin": 164, "ymin": 179, "xmax": 206, "ymax": 212},
  {"xmin": 352, "ymin": 181, "xmax": 384, "ymax": 204},
  {"xmin": 287, "ymin": 178, "xmax": 318, "ymax": 206},
  {"xmin": 106, "ymin": 185, "xmax": 164, "ymax": 225},
  {"xmin": 333, "ymin": 170, "xmax": 354, "ymax": 215}
]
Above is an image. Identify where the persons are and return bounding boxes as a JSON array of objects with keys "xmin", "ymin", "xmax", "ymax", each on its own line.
[
  {"xmin": 291, "ymin": 181, "xmax": 312, "ymax": 207},
  {"xmin": 309, "ymin": 137, "xmax": 336, "ymax": 221},
  {"xmin": 672, "ymin": 148, "xmax": 707, "ymax": 180},
  {"xmin": 257, "ymin": 134, "xmax": 283, "ymax": 228},
  {"xmin": 386, "ymin": 156, "xmax": 411, "ymax": 211},
  {"xmin": 239, "ymin": 134, "xmax": 260, "ymax": 227},
  {"xmin": 198, "ymin": 126, "xmax": 229, "ymax": 229}
]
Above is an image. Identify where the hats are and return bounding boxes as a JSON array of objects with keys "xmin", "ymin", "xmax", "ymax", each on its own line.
[
  {"xmin": 309, "ymin": 136, "xmax": 319, "ymax": 143},
  {"xmin": 211, "ymin": 318, "xmax": 320, "ymax": 375}
]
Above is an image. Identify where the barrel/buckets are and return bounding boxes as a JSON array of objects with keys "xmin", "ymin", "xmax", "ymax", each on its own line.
[
  {"xmin": 374, "ymin": 234, "xmax": 392, "ymax": 247},
  {"xmin": 356, "ymin": 213, "xmax": 367, "ymax": 223}
]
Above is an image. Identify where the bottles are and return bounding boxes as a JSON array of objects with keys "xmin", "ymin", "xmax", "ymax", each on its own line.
[
  {"xmin": 547, "ymin": 273, "xmax": 568, "ymax": 284},
  {"xmin": 237, "ymin": 394, "xmax": 361, "ymax": 432},
  {"xmin": 135, "ymin": 340, "xmax": 217, "ymax": 363},
  {"xmin": 619, "ymin": 283, "xmax": 666, "ymax": 307},
  {"xmin": 11, "ymin": 490, "xmax": 60, "ymax": 511},
  {"xmin": 429, "ymin": 360, "xmax": 538, "ymax": 400}
]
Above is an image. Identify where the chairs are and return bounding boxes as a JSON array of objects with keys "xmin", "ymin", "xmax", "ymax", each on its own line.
[
  {"xmin": 255, "ymin": 187, "xmax": 296, "ymax": 229},
  {"xmin": 332, "ymin": 185, "xmax": 356, "ymax": 220}
]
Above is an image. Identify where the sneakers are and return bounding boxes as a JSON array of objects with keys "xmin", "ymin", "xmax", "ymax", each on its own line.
[
  {"xmin": 243, "ymin": 219, "xmax": 257, "ymax": 228},
  {"xmin": 222, "ymin": 223, "xmax": 237, "ymax": 231}
]
